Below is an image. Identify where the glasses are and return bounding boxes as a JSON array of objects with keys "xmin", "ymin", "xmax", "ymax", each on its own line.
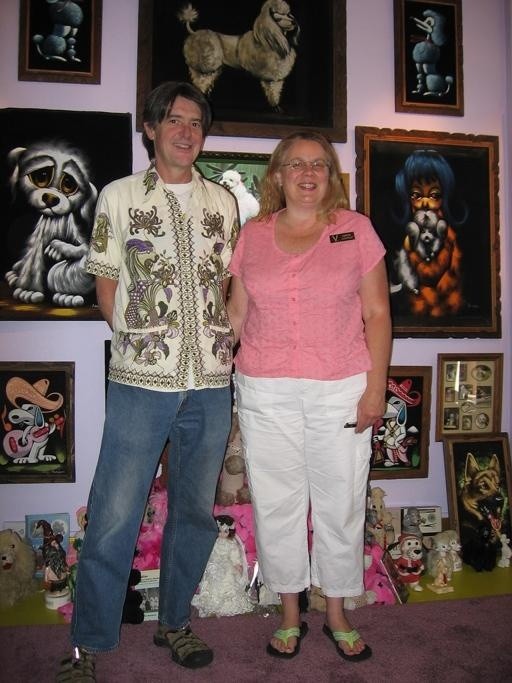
[{"xmin": 282, "ymin": 158, "xmax": 329, "ymax": 170}]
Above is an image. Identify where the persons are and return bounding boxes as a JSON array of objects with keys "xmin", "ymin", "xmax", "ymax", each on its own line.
[
  {"xmin": 53, "ymin": 81, "xmax": 239, "ymax": 683},
  {"xmin": 196, "ymin": 513, "xmax": 254, "ymax": 617},
  {"xmin": 226, "ymin": 128, "xmax": 391, "ymax": 663}
]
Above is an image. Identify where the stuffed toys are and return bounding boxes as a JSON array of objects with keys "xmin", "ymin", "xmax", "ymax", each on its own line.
[
  {"xmin": 364, "ymin": 486, "xmax": 393, "ymax": 547},
  {"xmin": 44, "ymin": 536, "xmax": 71, "ymax": 612},
  {"xmin": 0, "ymin": 529, "xmax": 35, "ymax": 608},
  {"xmin": 394, "ymin": 529, "xmax": 424, "ymax": 592},
  {"xmin": 444, "ymin": 529, "xmax": 461, "ymax": 572},
  {"xmin": 427, "ymin": 533, "xmax": 453, "ymax": 595},
  {"xmin": 401, "ymin": 508, "xmax": 422, "ymax": 540}
]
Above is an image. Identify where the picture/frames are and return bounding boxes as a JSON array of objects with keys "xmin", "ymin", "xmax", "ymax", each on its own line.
[
  {"xmin": 193, "ymin": 150, "xmax": 273, "ymax": 165},
  {"xmin": 392, "ymin": 0, "xmax": 465, "ymax": 116},
  {"xmin": 0, "ymin": 108, "xmax": 133, "ymax": 320},
  {"xmin": 0, "ymin": 360, "xmax": 76, "ymax": 484},
  {"xmin": 435, "ymin": 352, "xmax": 504, "ymax": 442},
  {"xmin": 442, "ymin": 431, "xmax": 512, "ymax": 555},
  {"xmin": 367, "ymin": 365, "xmax": 433, "ymax": 479},
  {"xmin": 17, "ymin": 0, "xmax": 102, "ymax": 84},
  {"xmin": 136, "ymin": 0, "xmax": 348, "ymax": 143},
  {"xmin": 104, "ymin": 339, "xmax": 114, "ymax": 415},
  {"xmin": 355, "ymin": 125, "xmax": 503, "ymax": 339}
]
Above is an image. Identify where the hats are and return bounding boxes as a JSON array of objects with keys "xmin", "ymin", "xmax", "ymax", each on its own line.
[
  {"xmin": 6, "ymin": 377, "xmax": 64, "ymax": 413},
  {"xmin": 387, "ymin": 378, "xmax": 421, "ymax": 408}
]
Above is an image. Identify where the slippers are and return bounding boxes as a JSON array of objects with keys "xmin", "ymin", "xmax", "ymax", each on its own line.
[
  {"xmin": 267, "ymin": 621, "xmax": 308, "ymax": 657},
  {"xmin": 323, "ymin": 620, "xmax": 372, "ymax": 661}
]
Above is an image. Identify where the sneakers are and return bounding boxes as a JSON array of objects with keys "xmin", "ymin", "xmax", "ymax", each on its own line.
[
  {"xmin": 152, "ymin": 623, "xmax": 212, "ymax": 668},
  {"xmin": 56, "ymin": 647, "xmax": 95, "ymax": 683}
]
